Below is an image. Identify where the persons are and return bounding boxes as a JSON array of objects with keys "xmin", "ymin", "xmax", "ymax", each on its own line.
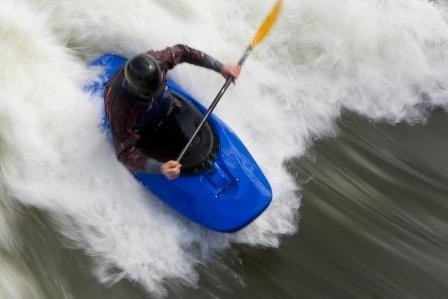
[{"xmin": 103, "ymin": 44, "xmax": 242, "ymax": 182}]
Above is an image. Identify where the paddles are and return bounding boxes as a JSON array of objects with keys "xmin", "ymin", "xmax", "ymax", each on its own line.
[{"xmin": 177, "ymin": 1, "xmax": 283, "ymax": 162}]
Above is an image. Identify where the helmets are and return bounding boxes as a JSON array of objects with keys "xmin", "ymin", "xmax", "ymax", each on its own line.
[{"xmin": 125, "ymin": 52, "xmax": 165, "ymax": 99}]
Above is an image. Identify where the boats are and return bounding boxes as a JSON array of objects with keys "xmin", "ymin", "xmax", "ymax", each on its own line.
[{"xmin": 83, "ymin": 53, "xmax": 272, "ymax": 233}]
